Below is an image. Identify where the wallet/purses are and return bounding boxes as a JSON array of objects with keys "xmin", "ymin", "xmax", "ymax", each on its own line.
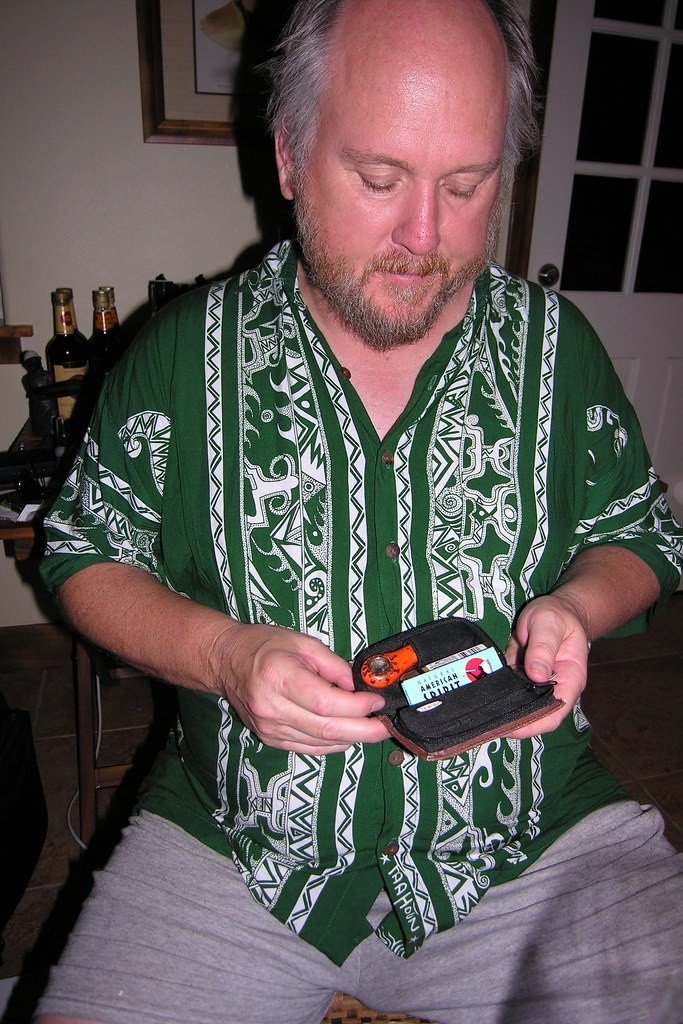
[{"xmin": 350, "ymin": 617, "xmax": 566, "ymax": 761}]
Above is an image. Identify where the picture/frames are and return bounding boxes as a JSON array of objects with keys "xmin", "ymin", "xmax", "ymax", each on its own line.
[{"xmin": 137, "ymin": 1, "xmax": 264, "ymax": 144}]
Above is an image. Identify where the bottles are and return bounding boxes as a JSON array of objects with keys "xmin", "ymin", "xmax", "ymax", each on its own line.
[{"xmin": 47, "ymin": 287, "xmax": 119, "ymax": 425}]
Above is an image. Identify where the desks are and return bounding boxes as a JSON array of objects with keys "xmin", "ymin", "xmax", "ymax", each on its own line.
[{"xmin": 0, "ymin": 412, "xmax": 160, "ymax": 850}]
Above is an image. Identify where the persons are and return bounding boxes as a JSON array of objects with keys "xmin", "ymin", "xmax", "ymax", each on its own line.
[{"xmin": 27, "ymin": 0, "xmax": 683, "ymax": 1024}]
